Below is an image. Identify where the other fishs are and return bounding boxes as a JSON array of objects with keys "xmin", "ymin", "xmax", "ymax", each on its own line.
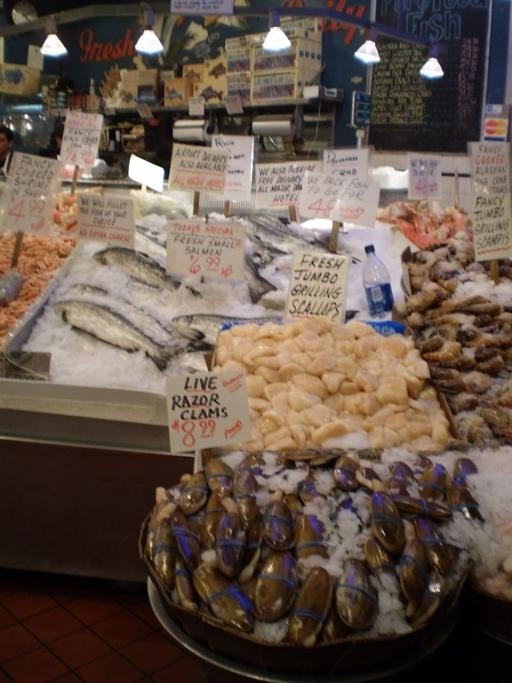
[
  {"xmin": 53, "ymin": 301, "xmax": 171, "ymax": 370},
  {"xmin": 92, "ymin": 247, "xmax": 203, "ymax": 298},
  {"xmin": 249, "ymin": 215, "xmax": 360, "ymax": 265},
  {"xmin": 246, "ymin": 232, "xmax": 275, "ymax": 266},
  {"xmin": 133, "ymin": 223, "xmax": 277, "ymax": 304},
  {"xmin": 172, "ymin": 315, "xmax": 285, "ymax": 348},
  {"xmin": 72, "ymin": 283, "xmax": 193, "ymax": 354}
]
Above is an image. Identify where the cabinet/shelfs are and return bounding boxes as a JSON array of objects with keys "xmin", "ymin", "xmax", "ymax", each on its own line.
[
  {"xmin": 48, "ymin": 96, "xmax": 334, "ymax": 165},
  {"xmin": 1, "ymin": 177, "xmax": 455, "ymax": 589}
]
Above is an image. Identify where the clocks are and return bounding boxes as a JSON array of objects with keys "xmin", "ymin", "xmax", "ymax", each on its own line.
[{"xmin": 9, "ymin": 0, "xmax": 37, "ymax": 27}]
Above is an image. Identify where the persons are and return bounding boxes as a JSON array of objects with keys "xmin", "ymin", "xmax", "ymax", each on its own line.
[{"xmin": 1, "ymin": 125, "xmax": 14, "ymax": 176}]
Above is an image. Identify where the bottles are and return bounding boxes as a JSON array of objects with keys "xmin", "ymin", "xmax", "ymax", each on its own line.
[{"xmin": 360, "ymin": 243, "xmax": 395, "ymax": 319}]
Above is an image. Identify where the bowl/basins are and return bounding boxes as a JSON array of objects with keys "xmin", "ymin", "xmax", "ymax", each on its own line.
[{"xmin": 136, "ymin": 516, "xmax": 467, "ymax": 683}]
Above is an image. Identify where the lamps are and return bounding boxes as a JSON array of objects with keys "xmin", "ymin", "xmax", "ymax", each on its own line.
[
  {"xmin": 134, "ymin": 10, "xmax": 165, "ymax": 54},
  {"xmin": 40, "ymin": 16, "xmax": 68, "ymax": 56},
  {"xmin": 353, "ymin": 28, "xmax": 380, "ymax": 63},
  {"xmin": 420, "ymin": 45, "xmax": 445, "ymax": 79},
  {"xmin": 262, "ymin": 10, "xmax": 291, "ymax": 51}
]
